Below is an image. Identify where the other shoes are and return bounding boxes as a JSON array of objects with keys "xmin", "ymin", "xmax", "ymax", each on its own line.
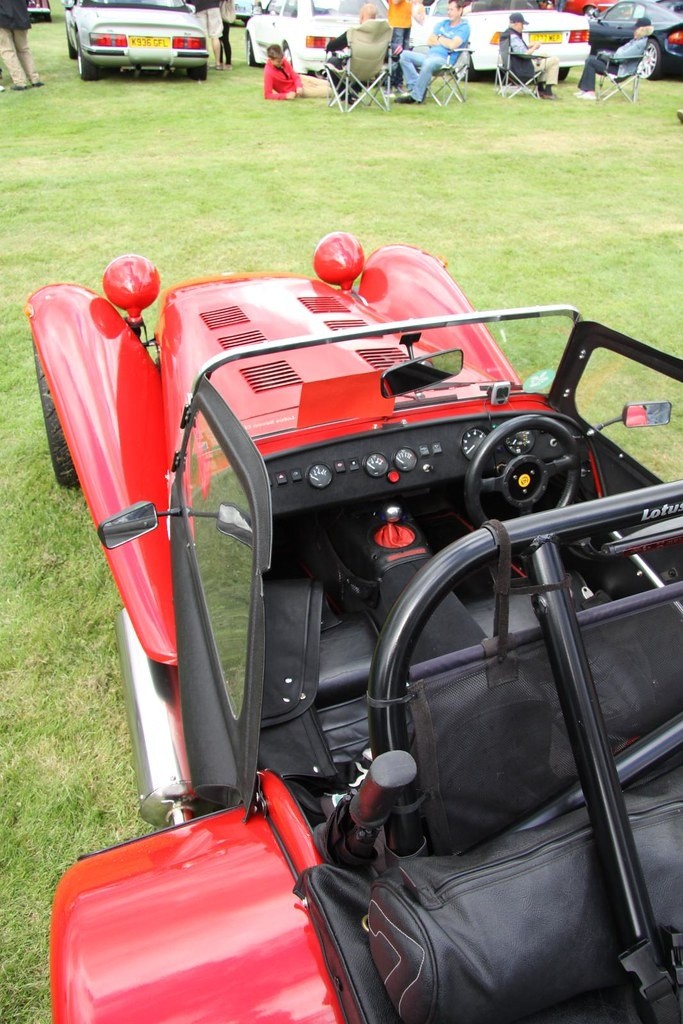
[
  {"xmin": 394, "ymin": 96, "xmax": 416, "ymax": 104},
  {"xmin": 572, "ymin": 89, "xmax": 598, "ymax": 100},
  {"xmin": 384, "ymin": 89, "xmax": 395, "ymax": 98},
  {"xmin": 10, "ymin": 84, "xmax": 28, "ymax": 90},
  {"xmin": 32, "ymin": 82, "xmax": 45, "ymax": 87},
  {"xmin": 0, "ymin": 85, "xmax": 5, "ymax": 91},
  {"xmin": 535, "ymin": 90, "xmax": 545, "ymax": 97},
  {"xmin": 395, "ymin": 86, "xmax": 405, "ymax": 94},
  {"xmin": 216, "ymin": 64, "xmax": 222, "ymax": 70},
  {"xmin": 223, "ymin": 64, "xmax": 232, "ymax": 70},
  {"xmin": 542, "ymin": 92, "xmax": 559, "ymax": 100}
]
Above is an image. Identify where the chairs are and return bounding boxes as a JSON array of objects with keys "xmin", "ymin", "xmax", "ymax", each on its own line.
[
  {"xmin": 317, "ymin": 668, "xmax": 559, "ymax": 875},
  {"xmin": 493, "ymin": 33, "xmax": 549, "ymax": 98},
  {"xmin": 596, "ymin": 36, "xmax": 655, "ymax": 104},
  {"xmin": 325, "ymin": 19, "xmax": 477, "ymax": 113}
]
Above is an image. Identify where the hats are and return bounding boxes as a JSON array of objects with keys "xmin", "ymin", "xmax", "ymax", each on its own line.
[
  {"xmin": 631, "ymin": 17, "xmax": 651, "ymax": 30},
  {"xmin": 508, "ymin": 12, "xmax": 530, "ymax": 25}
]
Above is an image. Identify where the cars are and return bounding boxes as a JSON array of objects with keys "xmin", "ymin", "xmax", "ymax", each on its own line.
[
  {"xmin": 27, "ymin": 229, "xmax": 683, "ymax": 1024},
  {"xmin": 585, "ymin": 0, "xmax": 683, "ymax": 82},
  {"xmin": 28, "ymin": 0, "xmax": 53, "ymax": 22},
  {"xmin": 409, "ymin": 0, "xmax": 592, "ymax": 82},
  {"xmin": 231, "ymin": 0, "xmax": 254, "ymax": 27},
  {"xmin": 245, "ymin": 0, "xmax": 392, "ymax": 74},
  {"xmin": 60, "ymin": 0, "xmax": 208, "ymax": 80},
  {"xmin": 539, "ymin": 0, "xmax": 621, "ymax": 21}
]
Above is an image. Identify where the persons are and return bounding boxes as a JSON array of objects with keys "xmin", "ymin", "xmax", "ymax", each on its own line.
[
  {"xmin": 505, "ymin": 12, "xmax": 559, "ymax": 100},
  {"xmin": 187, "ymin": 0, "xmax": 236, "ymax": 70},
  {"xmin": 573, "ymin": 17, "xmax": 654, "ymax": 101},
  {"xmin": 263, "ymin": 44, "xmax": 331, "ymax": 100},
  {"xmin": 394, "ymin": 0, "xmax": 470, "ymax": 103},
  {"xmin": 327, "ymin": 4, "xmax": 400, "ymax": 106},
  {"xmin": 384, "ymin": 0, "xmax": 422, "ymax": 97},
  {"xmin": 0, "ymin": 0, "xmax": 44, "ymax": 90}
]
